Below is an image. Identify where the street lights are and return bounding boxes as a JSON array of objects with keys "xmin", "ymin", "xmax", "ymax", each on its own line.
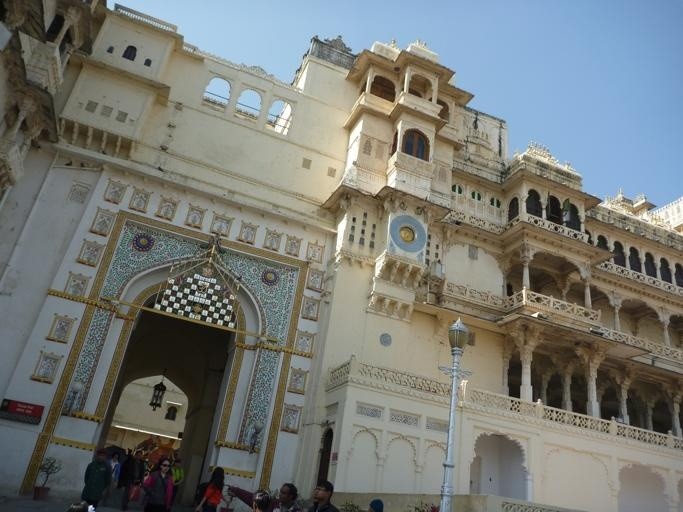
[{"xmin": 437, "ymin": 315, "xmax": 469, "ymax": 511}]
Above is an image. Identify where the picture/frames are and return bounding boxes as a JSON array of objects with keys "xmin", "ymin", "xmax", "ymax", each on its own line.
[
  {"xmin": 304, "ymin": 239, "xmax": 327, "ymax": 265},
  {"xmin": 126, "ymin": 184, "xmax": 155, "ymax": 215},
  {"xmin": 236, "ymin": 218, "xmax": 261, "ymax": 245},
  {"xmin": 29, "ymin": 349, "xmax": 66, "ymax": 387},
  {"xmin": 293, "ymin": 328, "xmax": 319, "ymax": 356},
  {"xmin": 63, "ymin": 269, "xmax": 94, "ymax": 299},
  {"xmin": 154, "ymin": 193, "xmax": 183, "ymax": 223},
  {"xmin": 209, "ymin": 211, "xmax": 236, "ymax": 238},
  {"xmin": 299, "ymin": 294, "xmax": 323, "ymax": 322},
  {"xmin": 278, "ymin": 400, "xmax": 305, "ymax": 435},
  {"xmin": 44, "ymin": 312, "xmax": 78, "ymax": 346},
  {"xmin": 263, "ymin": 226, "xmax": 286, "ymax": 252},
  {"xmin": 102, "ymin": 178, "xmax": 130, "ymax": 207},
  {"xmin": 305, "ymin": 267, "xmax": 325, "ymax": 294},
  {"xmin": 283, "ymin": 234, "xmax": 305, "ymax": 259},
  {"xmin": 182, "ymin": 202, "xmax": 210, "ymax": 229},
  {"xmin": 88, "ymin": 205, "xmax": 119, "ymax": 239},
  {"xmin": 74, "ymin": 236, "xmax": 108, "ymax": 269},
  {"xmin": 286, "ymin": 365, "xmax": 310, "ymax": 397}
]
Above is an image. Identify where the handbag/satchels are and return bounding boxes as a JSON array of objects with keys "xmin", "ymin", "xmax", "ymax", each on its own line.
[{"xmin": 129, "ymin": 485, "xmax": 140, "ymax": 501}]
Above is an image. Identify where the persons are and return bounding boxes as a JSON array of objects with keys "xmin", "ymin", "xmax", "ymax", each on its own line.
[
  {"xmin": 195, "ymin": 467, "xmax": 225, "ymax": 512},
  {"xmin": 369, "ymin": 499, "xmax": 384, "ymax": 512},
  {"xmin": 80, "ymin": 447, "xmax": 184, "ymax": 512},
  {"xmin": 252, "ymin": 480, "xmax": 339, "ymax": 512}
]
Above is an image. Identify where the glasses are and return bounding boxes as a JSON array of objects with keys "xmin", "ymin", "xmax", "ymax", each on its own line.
[{"xmin": 162, "ymin": 463, "xmax": 170, "ymax": 468}]
